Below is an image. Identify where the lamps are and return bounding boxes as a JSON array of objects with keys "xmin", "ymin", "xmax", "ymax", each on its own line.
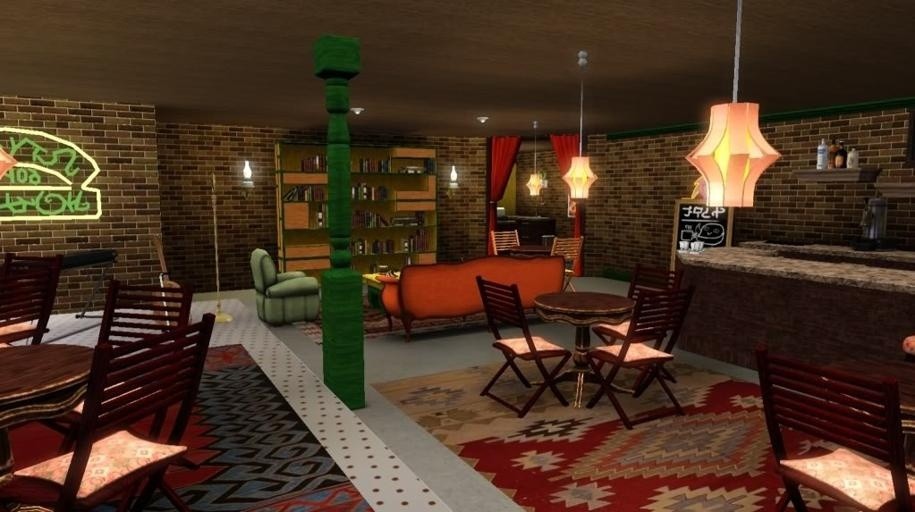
[
  {"xmin": 685, "ymin": 0, "xmax": 783, "ymax": 208},
  {"xmin": 561, "ymin": 50, "xmax": 599, "ymax": 199},
  {"xmin": 241, "ymin": 159, "xmax": 256, "ymax": 189},
  {"xmin": 525, "ymin": 120, "xmax": 544, "ymax": 196},
  {"xmin": 449, "ymin": 165, "xmax": 459, "ymax": 189}
]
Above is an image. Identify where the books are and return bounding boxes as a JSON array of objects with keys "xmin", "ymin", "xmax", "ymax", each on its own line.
[
  {"xmin": 350, "ymin": 156, "xmax": 429, "ymax": 274},
  {"xmin": 283, "ymin": 153, "xmax": 327, "ymax": 229}
]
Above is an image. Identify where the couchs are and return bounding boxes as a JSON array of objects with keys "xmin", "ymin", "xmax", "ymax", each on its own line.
[{"xmin": 378, "ymin": 255, "xmax": 566, "ymax": 342}]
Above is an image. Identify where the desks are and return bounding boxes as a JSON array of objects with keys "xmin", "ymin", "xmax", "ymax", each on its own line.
[
  {"xmin": 817, "ymin": 357, "xmax": 915, "ymax": 475},
  {"xmin": 511, "ymin": 245, "xmax": 551, "ymax": 257}
]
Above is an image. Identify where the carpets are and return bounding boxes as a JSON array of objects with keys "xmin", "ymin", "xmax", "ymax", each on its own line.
[
  {"xmin": 0, "ymin": 344, "xmax": 375, "ymax": 512},
  {"xmin": 291, "ymin": 296, "xmax": 543, "ymax": 345},
  {"xmin": 370, "ymin": 340, "xmax": 915, "ymax": 512}
]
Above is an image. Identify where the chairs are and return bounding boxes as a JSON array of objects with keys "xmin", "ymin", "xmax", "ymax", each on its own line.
[
  {"xmin": 249, "ymin": 248, "xmax": 320, "ymax": 324},
  {"xmin": 753, "ymin": 342, "xmax": 915, "ymax": 512},
  {"xmin": 591, "ymin": 262, "xmax": 687, "ymax": 390},
  {"xmin": 490, "ymin": 229, "xmax": 520, "ymax": 256},
  {"xmin": 0, "ymin": 249, "xmax": 216, "ymax": 512},
  {"xmin": 550, "ymin": 236, "xmax": 584, "ymax": 292},
  {"xmin": 476, "ymin": 275, "xmax": 572, "ymax": 418},
  {"xmin": 586, "ymin": 284, "xmax": 697, "ymax": 430}
]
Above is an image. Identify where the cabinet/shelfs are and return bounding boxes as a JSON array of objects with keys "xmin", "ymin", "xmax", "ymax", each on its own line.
[{"xmin": 274, "ymin": 140, "xmax": 438, "ymax": 288}]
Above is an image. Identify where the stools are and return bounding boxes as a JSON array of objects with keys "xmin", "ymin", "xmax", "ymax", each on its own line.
[{"xmin": 541, "ymin": 235, "xmax": 555, "ymax": 246}]
